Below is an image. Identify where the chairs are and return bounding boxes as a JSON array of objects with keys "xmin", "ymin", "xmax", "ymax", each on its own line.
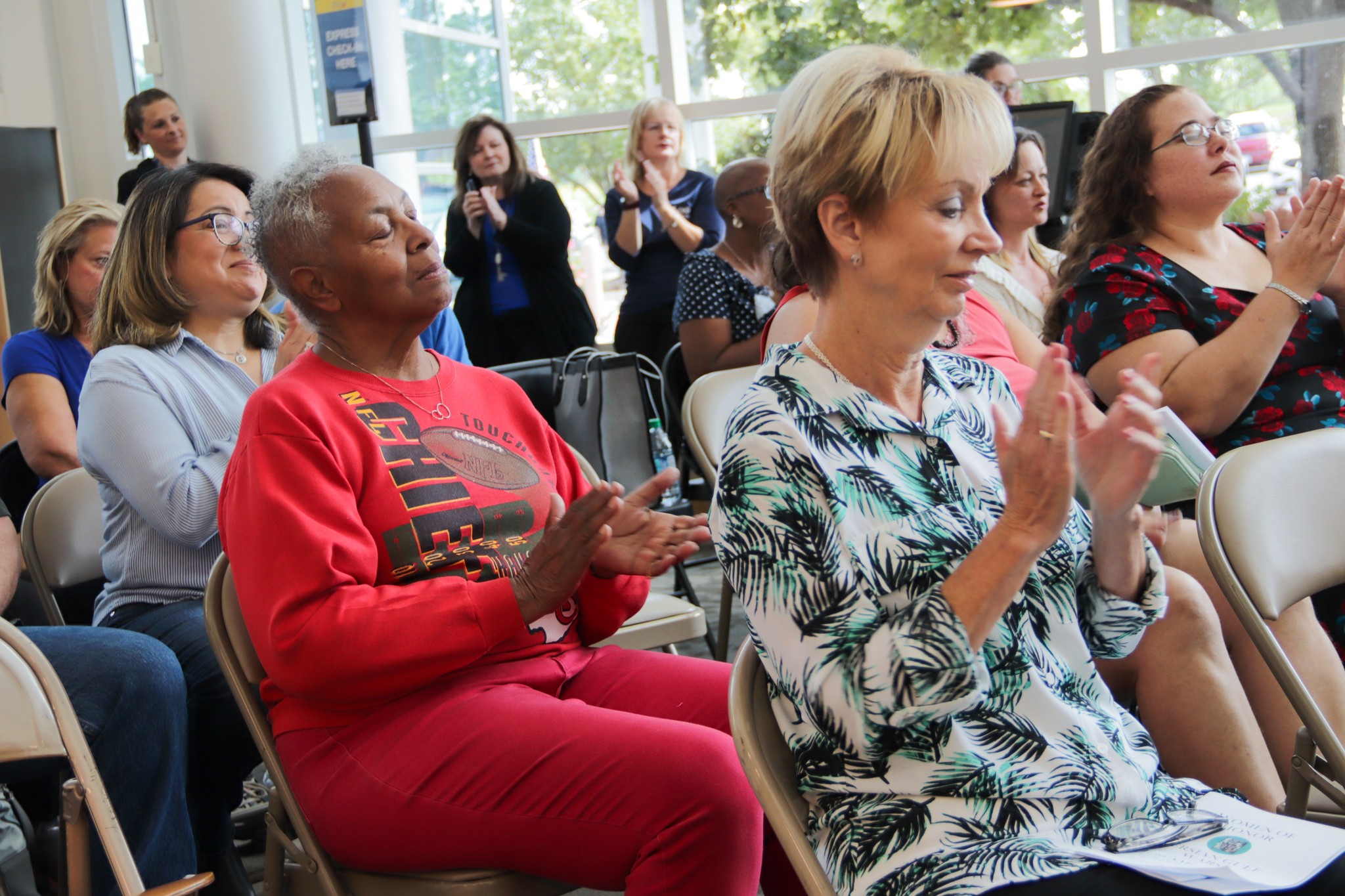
[
  {"xmin": 0, "ymin": 363, "xmax": 838, "ymax": 896},
  {"xmin": 1197, "ymin": 424, "xmax": 1345, "ymax": 819}
]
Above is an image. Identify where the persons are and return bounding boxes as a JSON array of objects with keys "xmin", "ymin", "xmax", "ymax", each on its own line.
[
  {"xmin": 969, "ymin": 126, "xmax": 1068, "ymax": 341},
  {"xmin": 0, "ymin": 196, "xmax": 129, "ymax": 626},
  {"xmin": 707, "ymin": 46, "xmax": 1345, "ymax": 896},
  {"xmin": 1041, "ymin": 84, "xmax": 1345, "ymax": 665},
  {"xmin": 926, "ymin": 285, "xmax": 1345, "ymax": 812},
  {"xmin": 268, "ymin": 286, "xmax": 473, "ymax": 365},
  {"xmin": 0, "ymin": 496, "xmax": 199, "ymax": 896},
  {"xmin": 74, "ymin": 161, "xmax": 319, "ymax": 896},
  {"xmin": 964, "ymin": 52, "xmax": 1022, "ymax": 106},
  {"xmin": 443, "ymin": 114, "xmax": 597, "ymax": 367},
  {"xmin": 672, "ymin": 158, "xmax": 783, "ymax": 385},
  {"xmin": 216, "ymin": 140, "xmax": 765, "ymax": 896},
  {"xmin": 605, "ymin": 93, "xmax": 725, "ymax": 460},
  {"xmin": 117, "ymin": 88, "xmax": 198, "ymax": 206}
]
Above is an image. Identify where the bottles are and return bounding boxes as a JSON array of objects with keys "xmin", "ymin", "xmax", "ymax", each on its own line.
[{"xmin": 647, "ymin": 418, "xmax": 682, "ymax": 507}]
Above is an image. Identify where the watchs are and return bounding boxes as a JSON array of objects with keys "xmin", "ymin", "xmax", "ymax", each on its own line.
[{"xmin": 1265, "ymin": 282, "xmax": 1312, "ymax": 314}]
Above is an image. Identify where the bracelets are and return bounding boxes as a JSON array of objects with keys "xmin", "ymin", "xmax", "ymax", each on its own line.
[
  {"xmin": 664, "ymin": 209, "xmax": 678, "ymax": 230},
  {"xmin": 621, "ymin": 199, "xmax": 641, "ymax": 209}
]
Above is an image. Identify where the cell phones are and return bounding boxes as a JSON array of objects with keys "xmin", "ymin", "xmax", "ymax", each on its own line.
[{"xmin": 465, "ymin": 179, "xmax": 480, "ymax": 218}]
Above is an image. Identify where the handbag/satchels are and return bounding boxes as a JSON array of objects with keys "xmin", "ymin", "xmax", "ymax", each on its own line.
[{"xmin": 550, "ymin": 346, "xmax": 662, "ymax": 512}]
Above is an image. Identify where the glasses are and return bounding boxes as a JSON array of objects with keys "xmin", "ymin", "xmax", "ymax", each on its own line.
[
  {"xmin": 1147, "ymin": 120, "xmax": 1239, "ymax": 159},
  {"xmin": 1101, "ymin": 809, "xmax": 1228, "ymax": 853},
  {"xmin": 728, "ymin": 184, "xmax": 772, "ymax": 202},
  {"xmin": 993, "ymin": 79, "xmax": 1025, "ymax": 98},
  {"xmin": 173, "ymin": 214, "xmax": 260, "ymax": 245}
]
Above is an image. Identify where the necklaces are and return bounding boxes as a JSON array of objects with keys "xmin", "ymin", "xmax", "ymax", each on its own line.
[
  {"xmin": 209, "ymin": 340, "xmax": 248, "ymax": 364},
  {"xmin": 932, "ymin": 319, "xmax": 959, "ymax": 348},
  {"xmin": 722, "ymin": 234, "xmax": 775, "ymax": 297}
]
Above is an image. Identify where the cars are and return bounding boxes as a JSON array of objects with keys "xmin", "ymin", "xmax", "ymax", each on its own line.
[{"xmin": 1226, "ymin": 113, "xmax": 1303, "ymax": 195}]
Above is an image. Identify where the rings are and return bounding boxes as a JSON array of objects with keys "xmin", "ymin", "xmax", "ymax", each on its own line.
[
  {"xmin": 304, "ymin": 341, "xmax": 314, "ymax": 351},
  {"xmin": 1039, "ymin": 429, "xmax": 1054, "ymax": 438}
]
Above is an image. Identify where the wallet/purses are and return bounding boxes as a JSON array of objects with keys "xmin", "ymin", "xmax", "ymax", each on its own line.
[{"xmin": 1074, "ymin": 440, "xmax": 1200, "ymax": 510}]
